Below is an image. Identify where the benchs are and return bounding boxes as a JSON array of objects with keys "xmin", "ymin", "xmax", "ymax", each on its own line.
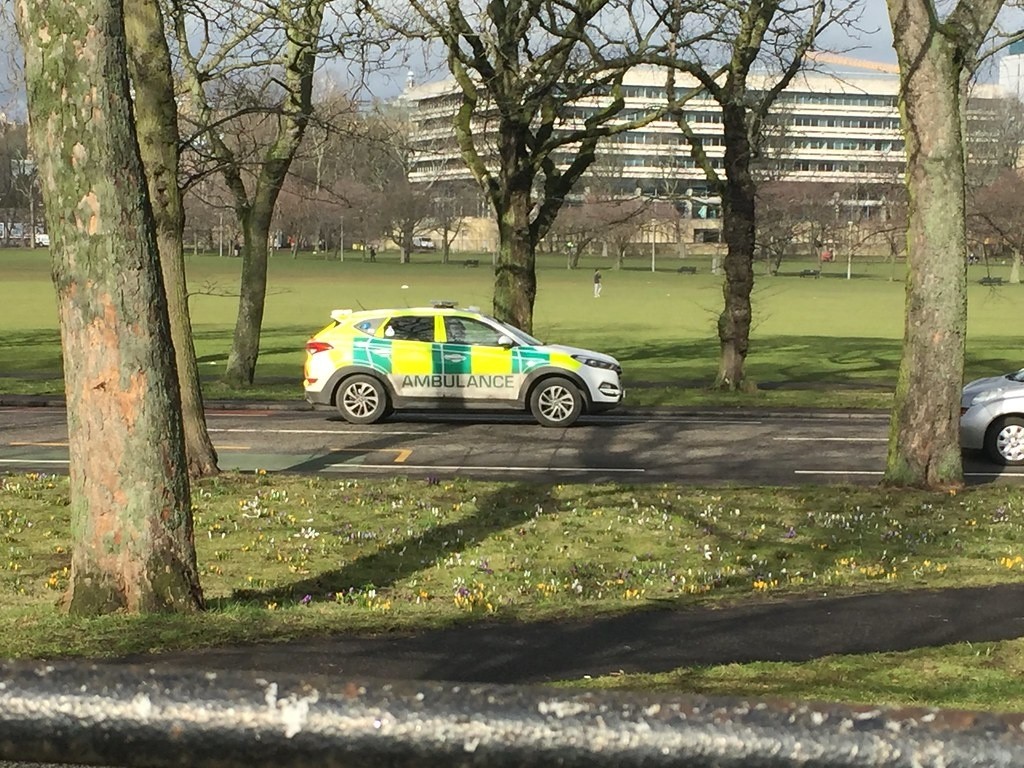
[
  {"xmin": 677, "ymin": 267, "xmax": 696, "ymax": 275},
  {"xmin": 464, "ymin": 260, "xmax": 480, "ymax": 267},
  {"xmin": 798, "ymin": 270, "xmax": 820, "ymax": 279},
  {"xmin": 977, "ymin": 277, "xmax": 1001, "ymax": 286}
]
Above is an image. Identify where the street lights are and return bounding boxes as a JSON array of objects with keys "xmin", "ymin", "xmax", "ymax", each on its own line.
[
  {"xmin": 651, "ymin": 218, "xmax": 656, "ymax": 272},
  {"xmin": 340, "ymin": 216, "xmax": 344, "ymax": 262},
  {"xmin": 847, "ymin": 221, "xmax": 853, "ymax": 279},
  {"xmin": 219, "ymin": 213, "xmax": 223, "ymax": 257}
]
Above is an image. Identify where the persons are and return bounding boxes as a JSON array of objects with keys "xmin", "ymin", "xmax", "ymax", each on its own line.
[
  {"xmin": 968, "ymin": 253, "xmax": 978, "ymax": 266},
  {"xmin": 370, "ymin": 247, "xmax": 376, "ymax": 262},
  {"xmin": 287, "ymin": 235, "xmax": 294, "ymax": 254},
  {"xmin": 593, "ymin": 268, "xmax": 602, "ymax": 297},
  {"xmin": 234, "ymin": 231, "xmax": 245, "ymax": 257}
]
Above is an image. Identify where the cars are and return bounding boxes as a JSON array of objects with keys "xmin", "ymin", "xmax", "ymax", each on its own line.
[
  {"xmin": 960, "ymin": 368, "xmax": 1024, "ymax": 466},
  {"xmin": 34, "ymin": 234, "xmax": 49, "ymax": 247},
  {"xmin": 412, "ymin": 237, "xmax": 434, "ymax": 248},
  {"xmin": 304, "ymin": 302, "xmax": 627, "ymax": 427}
]
